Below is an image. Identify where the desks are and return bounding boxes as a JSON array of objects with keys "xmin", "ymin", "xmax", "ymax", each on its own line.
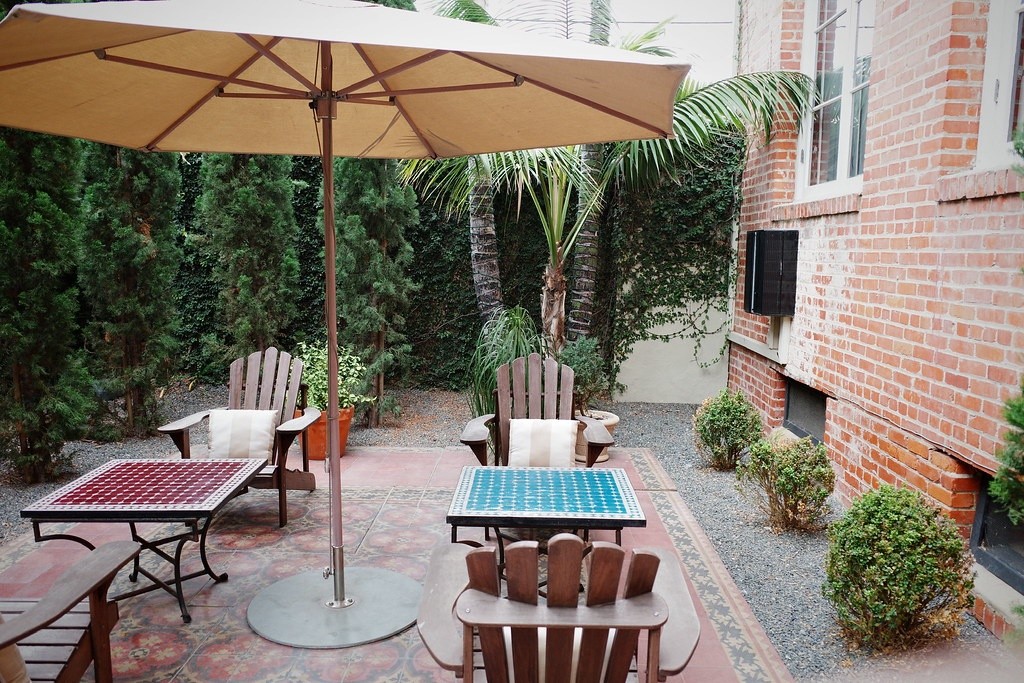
[
  {"xmin": 20, "ymin": 457, "xmax": 269, "ymax": 624},
  {"xmin": 446, "ymin": 465, "xmax": 648, "ymax": 598}
]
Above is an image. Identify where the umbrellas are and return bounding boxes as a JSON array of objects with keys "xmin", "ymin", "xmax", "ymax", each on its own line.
[{"xmin": 0, "ymin": 0, "xmax": 690, "ymax": 608}]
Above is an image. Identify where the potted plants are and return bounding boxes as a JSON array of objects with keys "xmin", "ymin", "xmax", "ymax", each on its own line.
[
  {"xmin": 556, "ymin": 333, "xmax": 620, "ymax": 462},
  {"xmin": 286, "ymin": 339, "xmax": 378, "ymax": 460}
]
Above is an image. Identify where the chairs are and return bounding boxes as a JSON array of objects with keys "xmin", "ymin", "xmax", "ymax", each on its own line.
[
  {"xmin": 0, "ymin": 539, "xmax": 141, "ymax": 683},
  {"xmin": 460, "ymin": 353, "xmax": 614, "ymax": 541},
  {"xmin": 157, "ymin": 346, "xmax": 321, "ymax": 528},
  {"xmin": 416, "ymin": 533, "xmax": 705, "ymax": 683}
]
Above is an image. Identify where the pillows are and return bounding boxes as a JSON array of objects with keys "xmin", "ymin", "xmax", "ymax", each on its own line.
[
  {"xmin": 0, "ymin": 615, "xmax": 32, "ymax": 683},
  {"xmin": 507, "ymin": 419, "xmax": 580, "ymax": 468},
  {"xmin": 207, "ymin": 410, "xmax": 278, "ymax": 467},
  {"xmin": 500, "ymin": 626, "xmax": 617, "ymax": 683}
]
